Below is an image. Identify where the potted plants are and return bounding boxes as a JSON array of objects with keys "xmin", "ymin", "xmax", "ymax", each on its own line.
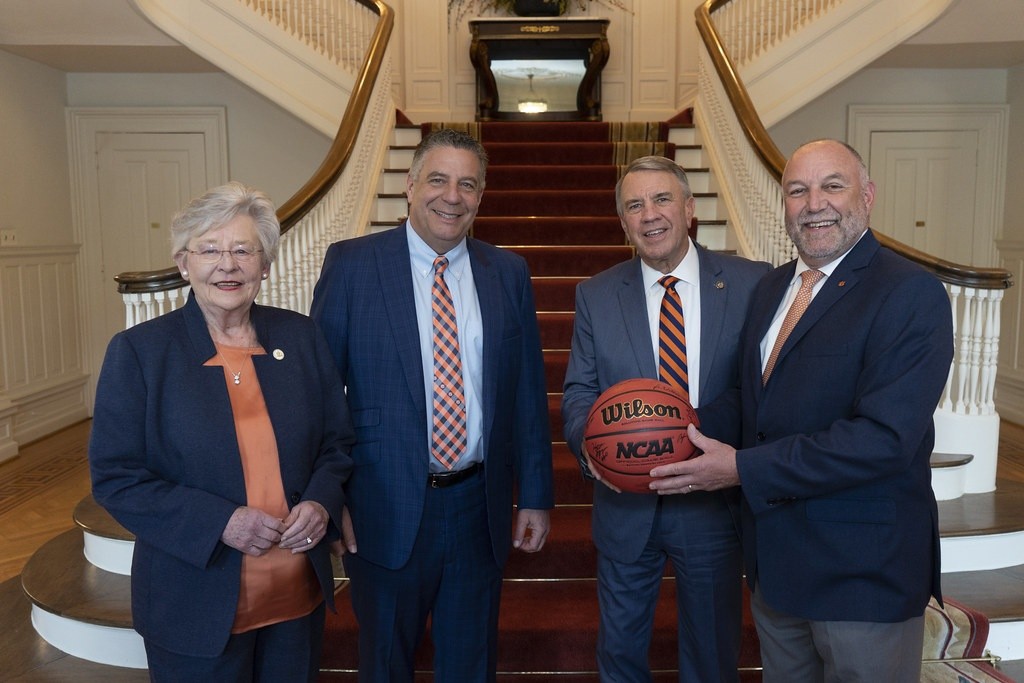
[{"xmin": 447, "ymin": 0, "xmax": 634, "ymax": 34}]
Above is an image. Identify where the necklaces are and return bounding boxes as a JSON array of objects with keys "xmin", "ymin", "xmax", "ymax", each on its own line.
[{"xmin": 199, "ymin": 322, "xmax": 258, "ymax": 385}]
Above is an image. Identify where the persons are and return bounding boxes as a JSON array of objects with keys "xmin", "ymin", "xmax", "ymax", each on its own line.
[
  {"xmin": 298, "ymin": 118, "xmax": 553, "ymax": 681},
  {"xmin": 649, "ymin": 136, "xmax": 954, "ymax": 682},
  {"xmin": 561, "ymin": 152, "xmax": 785, "ymax": 683},
  {"xmin": 82, "ymin": 182, "xmax": 356, "ymax": 681}
]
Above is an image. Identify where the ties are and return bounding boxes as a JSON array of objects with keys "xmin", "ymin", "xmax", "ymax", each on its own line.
[
  {"xmin": 762, "ymin": 269, "xmax": 826, "ymax": 388},
  {"xmin": 657, "ymin": 275, "xmax": 689, "ymax": 404},
  {"xmin": 432, "ymin": 256, "xmax": 467, "ymax": 471}
]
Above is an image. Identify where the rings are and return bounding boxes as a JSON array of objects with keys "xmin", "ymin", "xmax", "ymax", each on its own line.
[
  {"xmin": 687, "ymin": 484, "xmax": 694, "ymax": 494},
  {"xmin": 305, "ymin": 535, "xmax": 313, "ymax": 545}
]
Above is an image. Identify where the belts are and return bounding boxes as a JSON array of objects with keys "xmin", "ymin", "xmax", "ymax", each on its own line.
[{"xmin": 428, "ymin": 458, "xmax": 483, "ymax": 488}]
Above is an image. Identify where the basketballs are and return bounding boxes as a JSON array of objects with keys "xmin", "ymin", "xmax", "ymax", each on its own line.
[{"xmin": 584, "ymin": 376, "xmax": 701, "ymax": 495}]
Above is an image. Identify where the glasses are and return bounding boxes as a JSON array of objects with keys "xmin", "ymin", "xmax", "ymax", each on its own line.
[{"xmin": 183, "ymin": 243, "xmax": 265, "ymax": 264}]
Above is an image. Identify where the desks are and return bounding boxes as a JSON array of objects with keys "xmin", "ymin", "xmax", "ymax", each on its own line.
[{"xmin": 468, "ymin": 15, "xmax": 610, "ymax": 122}]
